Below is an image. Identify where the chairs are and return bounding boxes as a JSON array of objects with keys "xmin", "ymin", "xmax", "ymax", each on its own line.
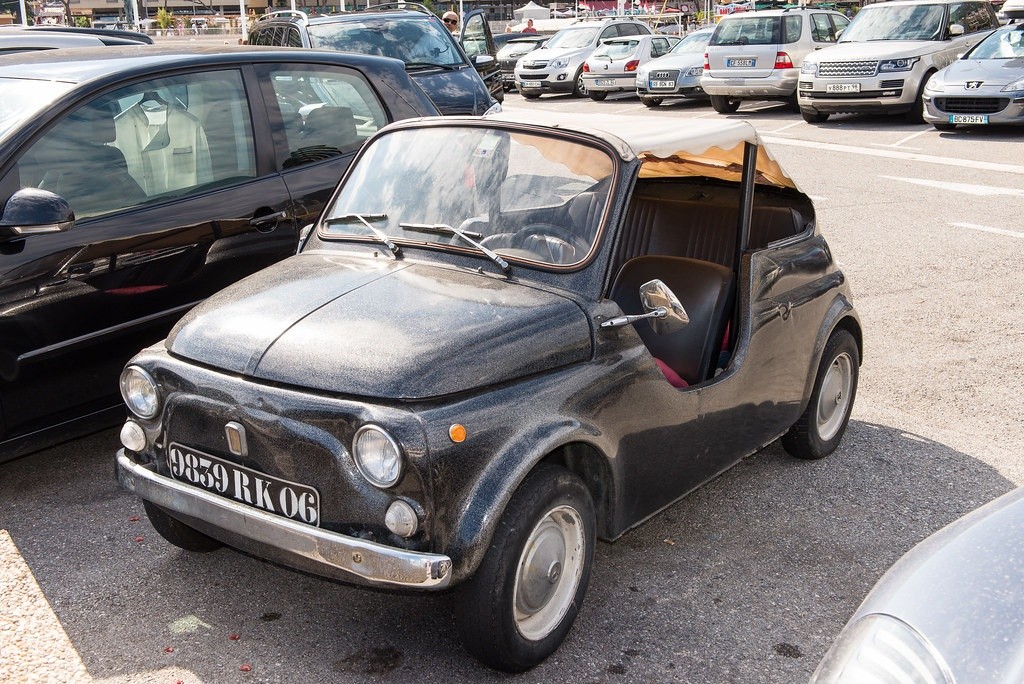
[
  {"xmin": 36, "ymin": 81, "xmax": 234, "ymax": 222},
  {"xmin": 276, "ymin": 102, "xmax": 360, "ymax": 155},
  {"xmin": 516, "ymin": 187, "xmax": 806, "ymax": 383}
]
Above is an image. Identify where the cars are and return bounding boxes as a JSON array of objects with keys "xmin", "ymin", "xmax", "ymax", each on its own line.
[
  {"xmin": 109, "ymin": 108, "xmax": 865, "ymax": 674},
  {"xmin": 462, "ymin": 33, "xmax": 543, "ymax": 61},
  {"xmin": 496, "ymin": 35, "xmax": 553, "ymax": 93},
  {"xmin": 920, "ymin": 22, "xmax": 1024, "ymax": 131}
]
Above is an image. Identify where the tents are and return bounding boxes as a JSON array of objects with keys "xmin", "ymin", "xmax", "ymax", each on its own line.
[
  {"xmin": 513, "ymin": 1, "xmax": 550, "ymax": 19},
  {"xmin": 587, "ymin": 0, "xmax": 631, "ymax": 12}
]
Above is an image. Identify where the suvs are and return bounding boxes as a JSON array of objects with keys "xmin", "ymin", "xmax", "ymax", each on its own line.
[
  {"xmin": 797, "ymin": 0, "xmax": 1003, "ymax": 125},
  {"xmin": 245, "ymin": 2, "xmax": 511, "ymax": 196},
  {"xmin": 582, "ymin": 34, "xmax": 684, "ymax": 101},
  {"xmin": 635, "ymin": 22, "xmax": 793, "ymax": 115},
  {"xmin": 512, "ymin": 15, "xmax": 657, "ymax": 99},
  {"xmin": 0, "ymin": 22, "xmax": 152, "ymax": 57},
  {"xmin": 0, "ymin": 45, "xmax": 479, "ymax": 472},
  {"xmin": 701, "ymin": 6, "xmax": 852, "ymax": 115}
]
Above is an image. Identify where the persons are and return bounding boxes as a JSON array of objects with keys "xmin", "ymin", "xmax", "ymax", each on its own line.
[
  {"xmin": 178, "ymin": 20, "xmax": 209, "ymax": 35},
  {"xmin": 408, "ymin": 10, "xmax": 459, "ymax": 64},
  {"xmin": 242, "ymin": 40, "xmax": 247, "ymax": 44},
  {"xmin": 505, "ymin": 27, "xmax": 512, "ymax": 33},
  {"xmin": 521, "ymin": 19, "xmax": 537, "ymax": 33},
  {"xmin": 238, "ymin": 38, "xmax": 242, "ymax": 45}
]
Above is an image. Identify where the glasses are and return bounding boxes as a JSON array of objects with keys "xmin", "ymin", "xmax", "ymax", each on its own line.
[{"xmin": 442, "ymin": 18, "xmax": 457, "ymax": 25}]
